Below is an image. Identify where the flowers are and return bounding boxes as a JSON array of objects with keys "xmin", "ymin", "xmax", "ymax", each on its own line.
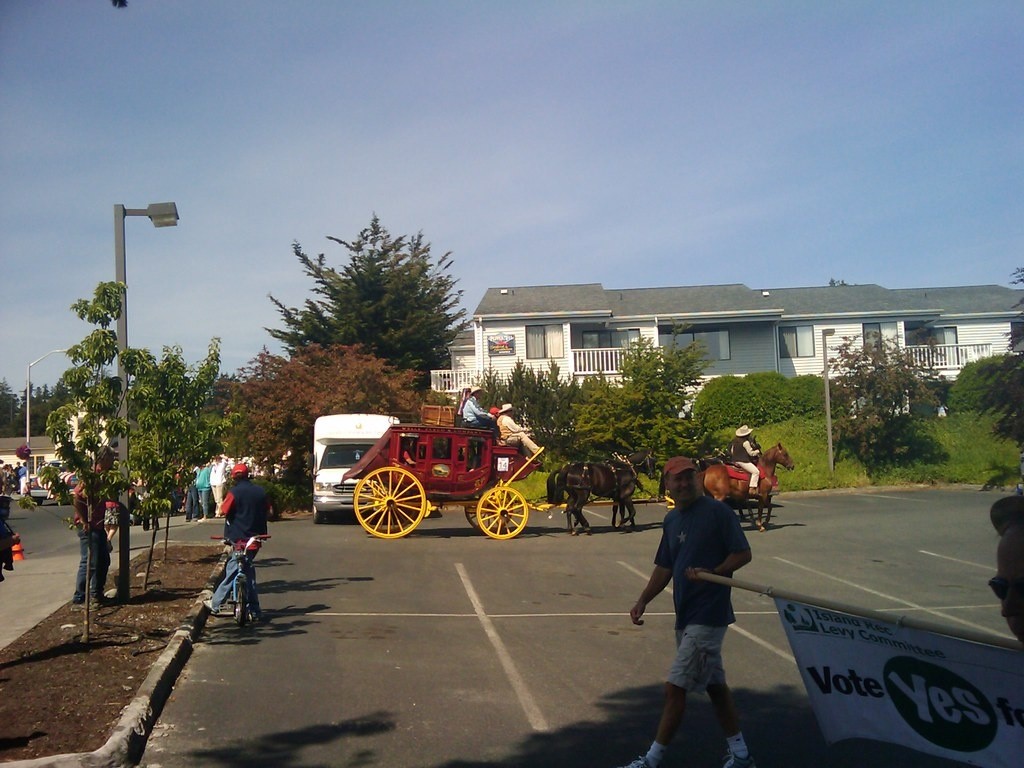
[{"xmin": 16, "ymin": 444, "xmax": 32, "ymax": 460}]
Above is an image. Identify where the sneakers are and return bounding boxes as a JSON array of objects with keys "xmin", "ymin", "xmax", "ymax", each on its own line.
[
  {"xmin": 71, "ymin": 594, "xmax": 116, "ymax": 612},
  {"xmin": 203, "ymin": 600, "xmax": 219, "ymax": 613},
  {"xmin": 722, "ymin": 748, "xmax": 756, "ymax": 768},
  {"xmin": 617, "ymin": 756, "xmax": 660, "ymax": 768}
]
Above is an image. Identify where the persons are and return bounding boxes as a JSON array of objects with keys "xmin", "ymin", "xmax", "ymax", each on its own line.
[
  {"xmin": 934, "ymin": 399, "xmax": 949, "ymax": 417},
  {"xmin": 73, "ymin": 445, "xmax": 119, "ymax": 603},
  {"xmin": 202, "ymin": 464, "xmax": 273, "ymax": 623},
  {"xmin": 834, "ymin": 404, "xmax": 856, "ymax": 419},
  {"xmin": 730, "ymin": 425, "xmax": 760, "ymax": 495},
  {"xmin": 631, "ymin": 457, "xmax": 752, "ymax": 768},
  {"xmin": 400, "ymin": 438, "xmax": 481, "ymax": 472},
  {"xmin": 142, "ymin": 454, "xmax": 237, "ymax": 531},
  {"xmin": 0, "ymin": 496, "xmax": 21, "ymax": 582},
  {"xmin": 0, "ymin": 461, "xmax": 75, "ymax": 496},
  {"xmin": 988, "ymin": 483, "xmax": 1024, "ymax": 645},
  {"xmin": 457, "ymin": 386, "xmax": 540, "ymax": 464}
]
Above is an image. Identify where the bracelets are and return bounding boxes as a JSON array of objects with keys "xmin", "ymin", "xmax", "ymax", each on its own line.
[{"xmin": 710, "ymin": 569, "xmax": 717, "ymax": 574}]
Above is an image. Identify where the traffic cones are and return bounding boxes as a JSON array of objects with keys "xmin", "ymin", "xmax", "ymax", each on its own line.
[{"xmin": 11, "ymin": 533, "xmax": 24, "ymax": 561}]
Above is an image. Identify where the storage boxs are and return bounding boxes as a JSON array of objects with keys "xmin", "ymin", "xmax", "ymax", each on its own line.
[{"xmin": 423, "ymin": 405, "xmax": 455, "ymax": 427}]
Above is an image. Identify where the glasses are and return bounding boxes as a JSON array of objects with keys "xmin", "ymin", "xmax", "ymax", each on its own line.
[{"xmin": 988, "ymin": 577, "xmax": 1024, "ymax": 600}]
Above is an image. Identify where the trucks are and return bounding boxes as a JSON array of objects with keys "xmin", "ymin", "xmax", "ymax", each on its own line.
[{"xmin": 313, "ymin": 415, "xmax": 401, "ymax": 524}]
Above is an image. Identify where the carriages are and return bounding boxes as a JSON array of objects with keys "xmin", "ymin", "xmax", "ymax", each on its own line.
[{"xmin": 341, "ymin": 427, "xmax": 658, "ymax": 541}]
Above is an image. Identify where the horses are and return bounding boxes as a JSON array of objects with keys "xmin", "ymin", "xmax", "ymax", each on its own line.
[
  {"xmin": 692, "ymin": 434, "xmax": 795, "ymax": 533},
  {"xmin": 546, "ymin": 447, "xmax": 656, "ymax": 537}
]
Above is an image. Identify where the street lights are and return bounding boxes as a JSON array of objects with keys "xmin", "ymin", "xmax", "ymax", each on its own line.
[
  {"xmin": 822, "ymin": 327, "xmax": 840, "ymax": 471},
  {"xmin": 114, "ymin": 200, "xmax": 180, "ymax": 601},
  {"xmin": 26, "ymin": 348, "xmax": 73, "ymax": 481}
]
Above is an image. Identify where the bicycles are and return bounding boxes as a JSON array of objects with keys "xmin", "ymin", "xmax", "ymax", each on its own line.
[{"xmin": 209, "ymin": 534, "xmax": 272, "ymax": 626}]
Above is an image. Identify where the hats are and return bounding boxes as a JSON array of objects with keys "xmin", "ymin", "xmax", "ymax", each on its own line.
[
  {"xmin": 499, "ymin": 404, "xmax": 515, "ymax": 414},
  {"xmin": 490, "ymin": 407, "xmax": 502, "ymax": 415},
  {"xmin": 232, "ymin": 464, "xmax": 248, "ymax": 478},
  {"xmin": 736, "ymin": 425, "xmax": 753, "ymax": 436},
  {"xmin": 470, "ymin": 385, "xmax": 481, "ymax": 394},
  {"xmin": 990, "ymin": 495, "xmax": 1024, "ymax": 537},
  {"xmin": 664, "ymin": 456, "xmax": 694, "ymax": 475}
]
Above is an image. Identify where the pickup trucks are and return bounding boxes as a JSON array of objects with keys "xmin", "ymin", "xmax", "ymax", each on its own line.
[{"xmin": 30, "ymin": 460, "xmax": 78, "ymax": 505}]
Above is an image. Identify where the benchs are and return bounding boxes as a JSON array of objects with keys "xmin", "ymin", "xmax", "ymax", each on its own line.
[{"xmin": 491, "ymin": 445, "xmax": 521, "ymax": 455}]
